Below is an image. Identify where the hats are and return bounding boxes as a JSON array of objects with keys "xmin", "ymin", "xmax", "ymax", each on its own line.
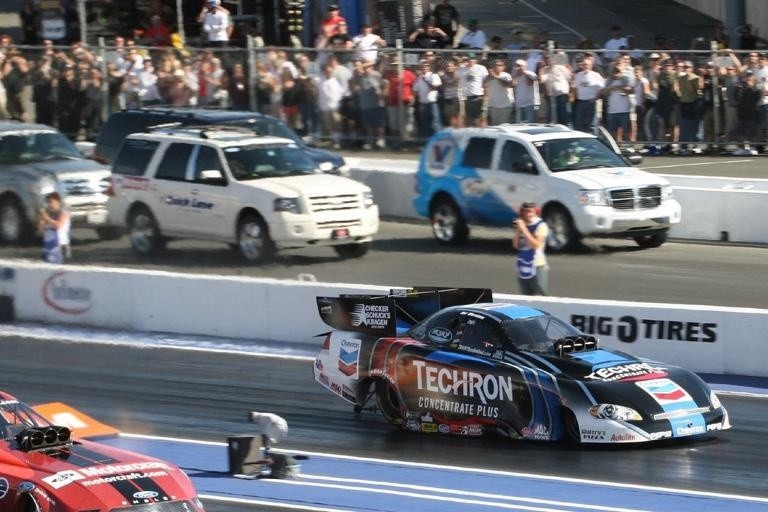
[{"xmin": 354, "ymin": 18, "xmax": 766, "ymax": 73}]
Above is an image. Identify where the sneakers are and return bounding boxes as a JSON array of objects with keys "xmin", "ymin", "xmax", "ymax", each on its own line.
[
  {"xmin": 625, "ymin": 140, "xmax": 767, "ymax": 155},
  {"xmin": 297, "ymin": 132, "xmax": 425, "ymax": 152}
]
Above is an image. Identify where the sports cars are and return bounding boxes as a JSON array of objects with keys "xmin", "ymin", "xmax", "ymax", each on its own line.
[
  {"xmin": 307, "ymin": 280, "xmax": 735, "ymax": 454},
  {"xmin": 0, "ymin": 387, "xmax": 208, "ymax": 512}
]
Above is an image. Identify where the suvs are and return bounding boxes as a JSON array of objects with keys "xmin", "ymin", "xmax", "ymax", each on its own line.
[
  {"xmin": 1, "ymin": 118, "xmax": 106, "ymax": 245},
  {"xmin": 95, "ymin": 107, "xmax": 348, "ymax": 183},
  {"xmin": 412, "ymin": 125, "xmax": 682, "ymax": 251},
  {"xmin": 105, "ymin": 125, "xmax": 381, "ymax": 266}
]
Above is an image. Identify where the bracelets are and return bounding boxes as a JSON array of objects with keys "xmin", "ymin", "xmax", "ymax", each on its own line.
[{"xmin": 45, "ymin": 217, "xmax": 51, "ymax": 224}]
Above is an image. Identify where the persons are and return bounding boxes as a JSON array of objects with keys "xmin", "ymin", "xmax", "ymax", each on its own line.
[
  {"xmin": 511, "ymin": 203, "xmax": 548, "ymax": 296},
  {"xmin": 558, "ymin": 145, "xmax": 579, "ymax": 166},
  {"xmin": 1, "ymin": 1, "xmax": 768, "ymax": 163},
  {"xmin": 38, "ymin": 191, "xmax": 72, "ymax": 264}
]
